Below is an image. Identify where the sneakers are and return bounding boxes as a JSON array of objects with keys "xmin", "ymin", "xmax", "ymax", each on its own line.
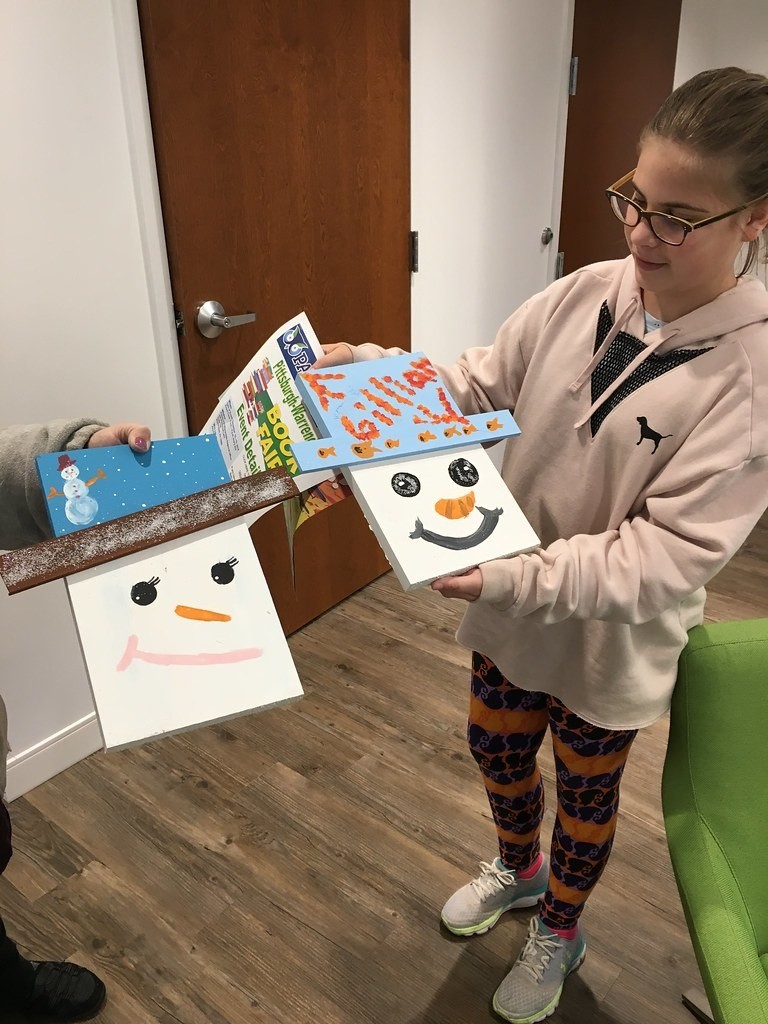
[
  {"xmin": 4, "ymin": 956, "xmax": 109, "ymax": 1024},
  {"xmin": 439, "ymin": 851, "xmax": 551, "ymax": 939},
  {"xmin": 490, "ymin": 916, "xmax": 588, "ymax": 1024}
]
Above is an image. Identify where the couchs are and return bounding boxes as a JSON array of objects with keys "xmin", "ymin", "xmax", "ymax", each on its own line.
[{"xmin": 657, "ymin": 618, "xmax": 766, "ymax": 1022}]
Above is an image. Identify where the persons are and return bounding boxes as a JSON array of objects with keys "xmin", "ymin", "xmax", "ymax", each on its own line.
[
  {"xmin": 312, "ymin": 68, "xmax": 768, "ymax": 1024},
  {"xmin": 0, "ymin": 418, "xmax": 150, "ymax": 1024}
]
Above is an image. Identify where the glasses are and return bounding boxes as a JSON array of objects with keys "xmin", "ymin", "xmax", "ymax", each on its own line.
[{"xmin": 602, "ymin": 164, "xmax": 767, "ymax": 250}]
